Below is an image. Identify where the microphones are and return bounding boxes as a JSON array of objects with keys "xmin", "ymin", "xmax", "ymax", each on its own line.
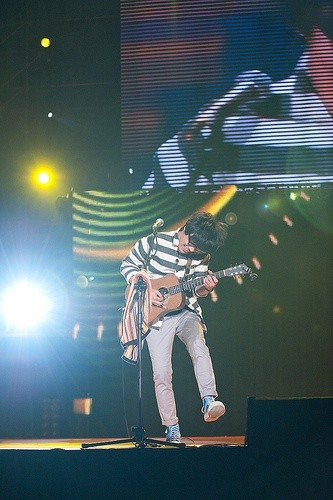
[
  {"xmin": 184, "ymin": 69, "xmax": 272, "ymax": 141},
  {"xmin": 152, "ymin": 217, "xmax": 164, "ymax": 229}
]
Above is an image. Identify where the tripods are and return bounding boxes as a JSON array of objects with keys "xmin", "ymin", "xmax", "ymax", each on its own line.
[{"xmin": 81, "ymin": 229, "xmax": 186, "ymax": 448}]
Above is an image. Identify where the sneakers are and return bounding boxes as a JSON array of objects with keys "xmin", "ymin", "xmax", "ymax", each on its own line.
[
  {"xmin": 200, "ymin": 395, "xmax": 226, "ymax": 423},
  {"xmin": 165, "ymin": 423, "xmax": 181, "ymax": 444}
]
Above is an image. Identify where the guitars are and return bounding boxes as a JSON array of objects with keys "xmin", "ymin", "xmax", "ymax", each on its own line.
[{"xmin": 125, "ymin": 264, "xmax": 258, "ymax": 327}]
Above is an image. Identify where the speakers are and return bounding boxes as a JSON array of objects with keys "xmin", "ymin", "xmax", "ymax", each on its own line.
[{"xmin": 246, "ymin": 395, "xmax": 333, "ymax": 445}]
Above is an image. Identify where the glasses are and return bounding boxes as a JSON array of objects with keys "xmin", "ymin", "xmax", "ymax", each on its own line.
[{"xmin": 187, "ymin": 234, "xmax": 207, "ymax": 253}]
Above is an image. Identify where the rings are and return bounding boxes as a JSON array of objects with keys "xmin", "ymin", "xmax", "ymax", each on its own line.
[{"xmin": 159, "ymin": 303, "xmax": 164, "ymax": 308}]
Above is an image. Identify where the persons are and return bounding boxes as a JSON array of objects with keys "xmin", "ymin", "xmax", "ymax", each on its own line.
[
  {"xmin": 120, "ymin": 212, "xmax": 226, "ymax": 444},
  {"xmin": 141, "ymin": 0, "xmax": 333, "ymax": 189}
]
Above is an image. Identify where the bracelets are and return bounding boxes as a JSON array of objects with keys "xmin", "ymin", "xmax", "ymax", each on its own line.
[{"xmin": 205, "ymin": 287, "xmax": 213, "ymax": 291}]
